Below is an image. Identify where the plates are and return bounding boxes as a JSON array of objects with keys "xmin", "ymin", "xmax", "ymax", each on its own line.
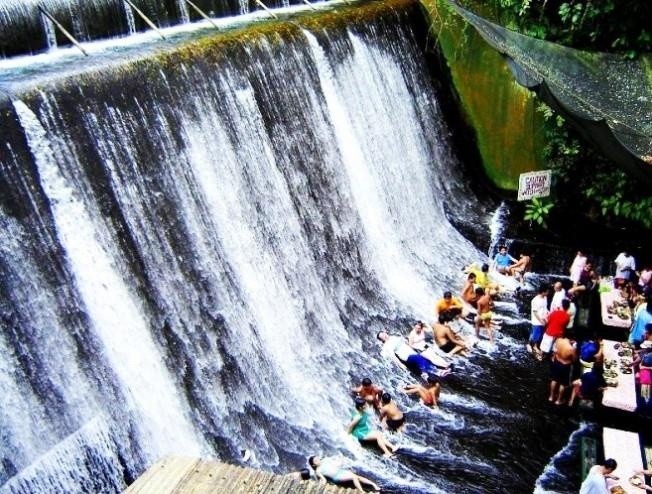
[
  {"xmin": 628, "ymin": 476, "xmax": 643, "ymax": 487},
  {"xmin": 606, "ymin": 300, "xmax": 629, "ymax": 319},
  {"xmin": 602, "ymin": 349, "xmax": 631, "ymax": 378}
]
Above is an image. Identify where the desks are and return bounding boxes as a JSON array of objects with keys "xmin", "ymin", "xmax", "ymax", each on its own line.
[
  {"xmin": 599, "ymin": 424, "xmax": 646, "ymax": 494},
  {"xmin": 600, "ymin": 336, "xmax": 639, "ymax": 416},
  {"xmin": 598, "ymin": 273, "xmax": 635, "ymax": 331}
]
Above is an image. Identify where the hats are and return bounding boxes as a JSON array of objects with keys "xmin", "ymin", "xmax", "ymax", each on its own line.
[{"xmin": 382, "ymin": 393, "xmax": 391, "ymax": 404}]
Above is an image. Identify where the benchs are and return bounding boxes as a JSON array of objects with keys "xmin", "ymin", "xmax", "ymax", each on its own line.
[
  {"xmin": 579, "ymin": 434, "xmax": 597, "ymax": 482},
  {"xmin": 577, "ymin": 306, "xmax": 590, "ymax": 328},
  {"xmin": 573, "ymin": 357, "xmax": 592, "ymax": 417}
]
